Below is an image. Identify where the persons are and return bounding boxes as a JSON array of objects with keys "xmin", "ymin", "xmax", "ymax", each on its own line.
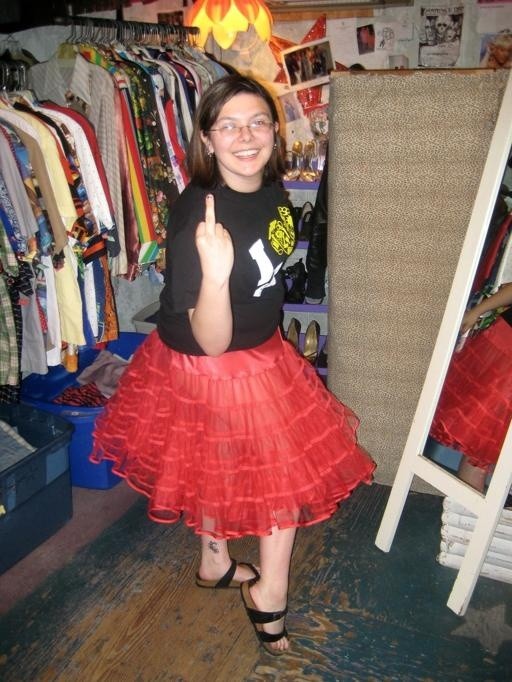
[
  {"xmin": 88, "ymin": 72, "xmax": 376, "ymax": 658},
  {"xmin": 428, "ymin": 282, "xmax": 512, "ymax": 490}
]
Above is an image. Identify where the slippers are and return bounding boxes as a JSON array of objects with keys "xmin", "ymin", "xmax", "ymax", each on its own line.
[
  {"xmin": 240, "ymin": 577, "xmax": 290, "ymax": 655},
  {"xmin": 196, "ymin": 559, "xmax": 260, "ymax": 589}
]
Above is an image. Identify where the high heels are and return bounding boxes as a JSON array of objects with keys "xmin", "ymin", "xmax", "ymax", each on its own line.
[{"xmin": 284, "ymin": 201, "xmax": 323, "ymax": 364}]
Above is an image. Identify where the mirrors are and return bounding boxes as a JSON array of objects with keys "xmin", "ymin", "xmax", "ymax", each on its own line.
[{"xmin": 371, "ymin": 74, "xmax": 512, "ymax": 615}]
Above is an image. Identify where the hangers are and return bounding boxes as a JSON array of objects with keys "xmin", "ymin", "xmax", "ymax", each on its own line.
[
  {"xmin": 57, "ymin": 15, "xmax": 199, "ymax": 69},
  {"xmin": 0, "ymin": 62, "xmax": 41, "ymax": 112}
]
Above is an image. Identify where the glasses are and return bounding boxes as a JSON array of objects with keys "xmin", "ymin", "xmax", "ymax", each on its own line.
[{"xmin": 204, "ymin": 120, "xmax": 276, "ymax": 137}]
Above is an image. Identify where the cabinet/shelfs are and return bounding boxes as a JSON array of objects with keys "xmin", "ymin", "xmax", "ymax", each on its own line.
[{"xmin": 278, "ymin": 178, "xmax": 330, "ymax": 378}]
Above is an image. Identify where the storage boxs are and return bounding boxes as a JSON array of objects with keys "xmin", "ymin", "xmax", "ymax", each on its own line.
[
  {"xmin": 3, "ymin": 402, "xmax": 76, "ymax": 572},
  {"xmin": 131, "ymin": 301, "xmax": 158, "ymax": 333},
  {"xmin": 19, "ymin": 331, "xmax": 148, "ymax": 490}
]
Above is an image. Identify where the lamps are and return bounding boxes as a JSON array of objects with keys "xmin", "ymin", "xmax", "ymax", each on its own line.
[{"xmin": 186, "ymin": 0, "xmax": 274, "ymax": 51}]
